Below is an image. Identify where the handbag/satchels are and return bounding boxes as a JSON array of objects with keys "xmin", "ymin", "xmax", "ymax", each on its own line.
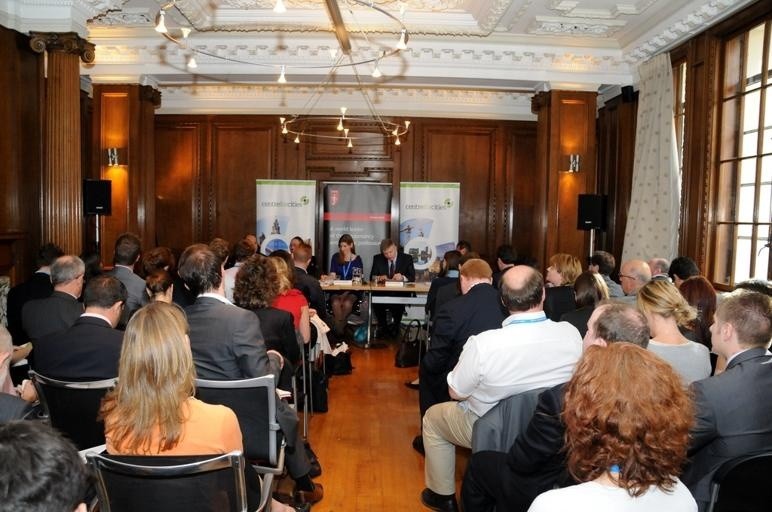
[
  {"xmin": 396, "ymin": 339, "xmax": 425, "ymax": 367},
  {"xmin": 299, "ymin": 366, "xmax": 328, "ymax": 412},
  {"xmin": 325, "ymin": 341, "xmax": 352, "ymax": 374},
  {"xmin": 304, "ymin": 442, "xmax": 320, "ymax": 478}
]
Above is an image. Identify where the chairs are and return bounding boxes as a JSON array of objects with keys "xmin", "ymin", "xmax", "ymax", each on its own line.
[
  {"xmin": 701, "ymin": 449, "xmax": 772, "ymax": 512},
  {"xmin": 25, "ymin": 371, "xmax": 289, "ymax": 511}
]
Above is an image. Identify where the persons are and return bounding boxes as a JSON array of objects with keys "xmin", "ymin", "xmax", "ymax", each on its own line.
[
  {"xmin": 329, "ymin": 233, "xmax": 364, "ymax": 348},
  {"xmin": 404, "ymin": 237, "xmax": 772, "ymax": 512},
  {"xmin": 1, "ymin": 233, "xmax": 333, "ymax": 512},
  {"xmin": 369, "ymin": 239, "xmax": 416, "ymax": 341}
]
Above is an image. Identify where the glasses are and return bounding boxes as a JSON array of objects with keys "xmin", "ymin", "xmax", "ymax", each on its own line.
[{"xmin": 618, "ymin": 272, "xmax": 635, "ymax": 280}]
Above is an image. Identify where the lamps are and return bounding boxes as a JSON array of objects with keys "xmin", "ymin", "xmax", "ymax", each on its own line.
[
  {"xmin": 106, "ymin": 148, "xmax": 118, "ymax": 167},
  {"xmin": 569, "ymin": 152, "xmax": 581, "ymax": 174},
  {"xmin": 279, "ymin": 53, "xmax": 411, "ymax": 148},
  {"xmin": 153, "ymin": 0, "xmax": 412, "ymax": 83}
]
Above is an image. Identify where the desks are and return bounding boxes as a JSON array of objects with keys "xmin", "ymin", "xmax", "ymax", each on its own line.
[{"xmin": 317, "ymin": 281, "xmax": 430, "ymax": 352}]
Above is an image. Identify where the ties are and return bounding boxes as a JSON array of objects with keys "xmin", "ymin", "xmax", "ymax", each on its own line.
[{"xmin": 390, "ymin": 260, "xmax": 396, "ymax": 278}]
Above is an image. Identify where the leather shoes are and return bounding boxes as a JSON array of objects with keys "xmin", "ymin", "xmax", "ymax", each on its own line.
[
  {"xmin": 294, "ymin": 483, "xmax": 323, "ymax": 510},
  {"xmin": 422, "ymin": 488, "xmax": 458, "ymax": 512},
  {"xmin": 405, "ymin": 380, "xmax": 420, "ymax": 389}
]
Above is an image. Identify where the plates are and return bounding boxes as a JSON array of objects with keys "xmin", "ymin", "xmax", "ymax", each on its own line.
[
  {"xmin": 385, "ymin": 282, "xmax": 403, "ymax": 287},
  {"xmin": 333, "ymin": 280, "xmax": 353, "ymax": 285}
]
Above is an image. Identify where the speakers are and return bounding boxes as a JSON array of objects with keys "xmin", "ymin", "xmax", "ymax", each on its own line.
[
  {"xmin": 83, "ymin": 179, "xmax": 111, "ymax": 216},
  {"xmin": 577, "ymin": 194, "xmax": 606, "ymax": 231}
]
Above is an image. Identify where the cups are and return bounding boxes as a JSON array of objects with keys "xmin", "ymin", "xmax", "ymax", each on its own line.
[
  {"xmin": 352, "ymin": 268, "xmax": 363, "ymax": 287},
  {"xmin": 372, "ymin": 275, "xmax": 381, "ymax": 285}
]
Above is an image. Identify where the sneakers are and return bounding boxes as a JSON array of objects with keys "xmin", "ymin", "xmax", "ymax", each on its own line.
[{"xmin": 413, "ymin": 435, "xmax": 424, "ymax": 455}]
